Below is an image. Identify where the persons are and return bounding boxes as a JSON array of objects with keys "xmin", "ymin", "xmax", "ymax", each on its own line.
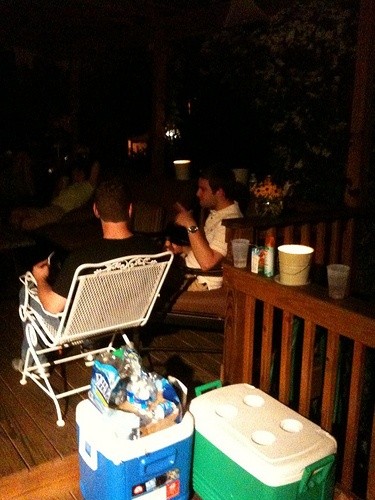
[
  {"xmin": 10, "ymin": 180, "xmax": 169, "ymax": 383},
  {"xmin": 158, "ymin": 160, "xmax": 246, "ymax": 313},
  {"xmin": 11, "ymin": 160, "xmax": 100, "ymax": 231}
]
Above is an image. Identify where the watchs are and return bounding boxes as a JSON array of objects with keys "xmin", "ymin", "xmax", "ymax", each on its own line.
[{"xmin": 187, "ymin": 224, "xmax": 200, "ymax": 233}]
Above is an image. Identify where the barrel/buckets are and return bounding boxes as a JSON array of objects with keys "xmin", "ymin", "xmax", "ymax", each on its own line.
[
  {"xmin": 173, "ymin": 160, "xmax": 191, "ymax": 180},
  {"xmin": 277, "ymin": 244, "xmax": 314, "ymax": 284},
  {"xmin": 232, "ymin": 169, "xmax": 247, "ymax": 186}
]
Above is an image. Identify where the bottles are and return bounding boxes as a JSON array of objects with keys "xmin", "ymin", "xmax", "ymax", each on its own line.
[
  {"xmin": 250, "ymin": 239, "xmax": 275, "ymax": 278},
  {"xmin": 248, "ymin": 173, "xmax": 257, "ymax": 188}
]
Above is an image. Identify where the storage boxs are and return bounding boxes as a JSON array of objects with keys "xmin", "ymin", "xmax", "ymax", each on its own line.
[
  {"xmin": 74, "ymin": 395, "xmax": 195, "ymax": 500},
  {"xmin": 189, "ymin": 378, "xmax": 340, "ymax": 500}
]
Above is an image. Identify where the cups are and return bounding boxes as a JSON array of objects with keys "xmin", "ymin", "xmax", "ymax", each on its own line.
[
  {"xmin": 230, "ymin": 238, "xmax": 250, "ymax": 268},
  {"xmin": 327, "ymin": 264, "xmax": 351, "ymax": 299}
]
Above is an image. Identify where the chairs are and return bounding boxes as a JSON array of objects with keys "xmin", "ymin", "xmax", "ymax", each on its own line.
[
  {"xmin": 138, "ymin": 265, "xmax": 228, "ymax": 353},
  {"xmin": 14, "ymin": 249, "xmax": 174, "ymax": 428}
]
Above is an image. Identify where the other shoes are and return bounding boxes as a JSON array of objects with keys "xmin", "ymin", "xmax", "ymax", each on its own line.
[
  {"xmin": 12, "ymin": 359, "xmax": 50, "ymax": 379},
  {"xmin": 80, "ymin": 344, "xmax": 98, "ymax": 367}
]
